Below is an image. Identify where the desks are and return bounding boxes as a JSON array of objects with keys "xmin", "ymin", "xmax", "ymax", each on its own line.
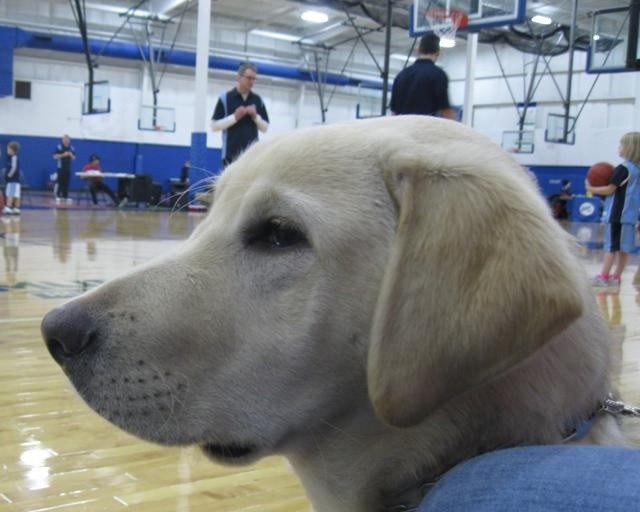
[{"xmin": 74, "ymin": 169, "xmax": 136, "ymax": 209}]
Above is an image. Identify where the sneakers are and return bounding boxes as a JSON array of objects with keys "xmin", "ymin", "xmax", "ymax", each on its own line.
[
  {"xmin": 590, "ymin": 286, "xmax": 620, "ymax": 296},
  {"xmin": 2, "ymin": 205, "xmax": 22, "ymax": 215},
  {"xmin": 590, "ymin": 274, "xmax": 620, "ymax": 287}
]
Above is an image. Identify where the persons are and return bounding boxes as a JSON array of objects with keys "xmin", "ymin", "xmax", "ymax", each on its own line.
[
  {"xmin": 390, "ymin": 33, "xmax": 457, "ymax": 120},
  {"xmin": 584, "ymin": 131, "xmax": 640, "ymax": 287},
  {"xmin": 1, "ymin": 214, "xmax": 20, "ymax": 284},
  {"xmin": 3, "ymin": 142, "xmax": 23, "ymax": 214},
  {"xmin": 210, "ymin": 64, "xmax": 271, "ymax": 166},
  {"xmin": 84, "ymin": 207, "xmax": 111, "ymax": 260},
  {"xmin": 53, "ymin": 133, "xmax": 77, "ymax": 201},
  {"xmin": 597, "ymin": 292, "xmax": 627, "ymax": 400},
  {"xmin": 51, "ymin": 206, "xmax": 72, "ymax": 266},
  {"xmin": 80, "ymin": 154, "xmax": 128, "ymax": 207},
  {"xmin": 555, "ymin": 180, "xmax": 574, "ymax": 231}
]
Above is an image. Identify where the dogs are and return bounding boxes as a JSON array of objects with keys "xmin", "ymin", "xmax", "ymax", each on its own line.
[{"xmin": 39, "ymin": 112, "xmax": 639, "ymax": 512}]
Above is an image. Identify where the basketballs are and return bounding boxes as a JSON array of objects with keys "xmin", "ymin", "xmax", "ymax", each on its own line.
[{"xmin": 586, "ymin": 162, "xmax": 614, "ymax": 187}]
